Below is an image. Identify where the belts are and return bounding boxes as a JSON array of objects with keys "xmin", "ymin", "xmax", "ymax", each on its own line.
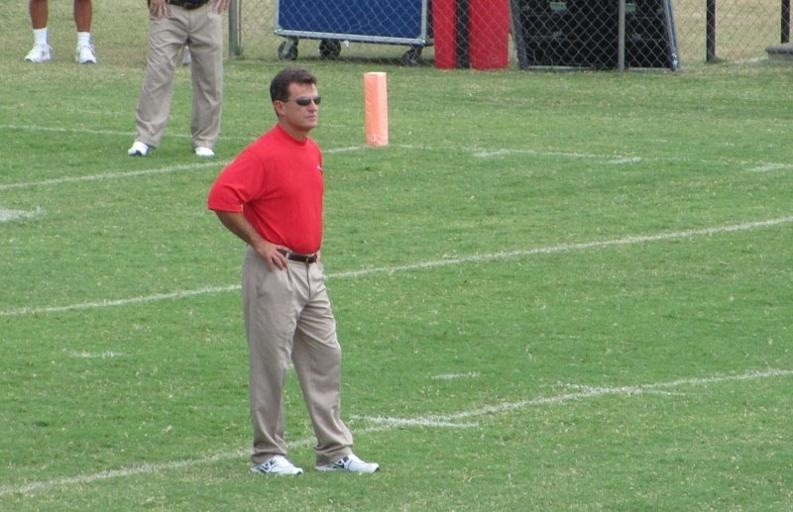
[
  {"xmin": 277, "ymin": 249, "xmax": 317, "ymax": 263},
  {"xmin": 166, "ymin": 0, "xmax": 207, "ymax": 10}
]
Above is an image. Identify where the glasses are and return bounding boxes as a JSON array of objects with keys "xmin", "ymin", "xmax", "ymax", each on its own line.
[{"xmin": 282, "ymin": 95, "xmax": 320, "ymax": 105}]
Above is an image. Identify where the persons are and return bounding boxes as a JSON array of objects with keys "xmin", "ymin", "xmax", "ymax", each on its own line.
[
  {"xmin": 177, "ymin": 41, "xmax": 193, "ymax": 66},
  {"xmin": 207, "ymin": 67, "xmax": 381, "ymax": 476},
  {"xmin": 21, "ymin": 1, "xmax": 93, "ymax": 67},
  {"xmin": 126, "ymin": 1, "xmax": 230, "ymax": 159}
]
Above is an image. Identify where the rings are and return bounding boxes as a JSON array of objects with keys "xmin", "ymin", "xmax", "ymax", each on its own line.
[{"xmin": 163, "ymin": 8, "xmax": 166, "ymax": 10}]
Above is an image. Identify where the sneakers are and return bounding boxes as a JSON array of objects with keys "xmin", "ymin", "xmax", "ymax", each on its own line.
[
  {"xmin": 194, "ymin": 146, "xmax": 214, "ymax": 157},
  {"xmin": 250, "ymin": 453, "xmax": 303, "ymax": 476},
  {"xmin": 74, "ymin": 45, "xmax": 96, "ymax": 64},
  {"xmin": 314, "ymin": 451, "xmax": 380, "ymax": 473},
  {"xmin": 127, "ymin": 141, "xmax": 149, "ymax": 156},
  {"xmin": 24, "ymin": 43, "xmax": 51, "ymax": 64}
]
